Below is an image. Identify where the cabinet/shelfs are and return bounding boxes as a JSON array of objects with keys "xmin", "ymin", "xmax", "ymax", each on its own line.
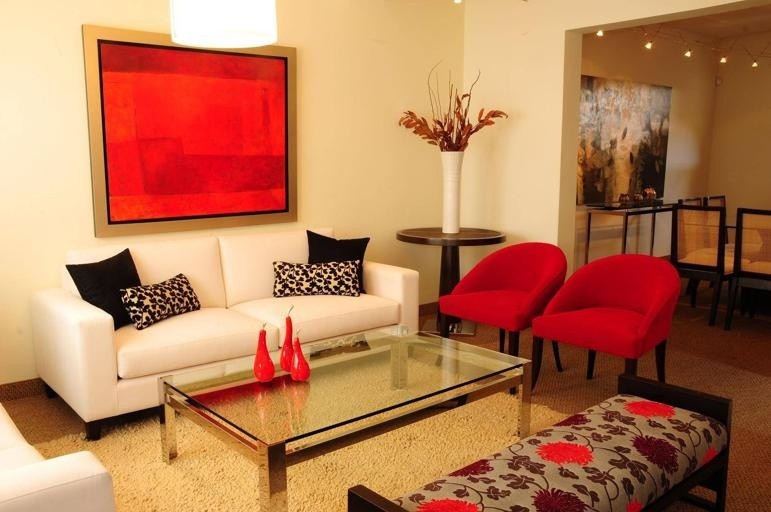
[{"xmin": 585, "ymin": 203, "xmax": 677, "ymax": 263}]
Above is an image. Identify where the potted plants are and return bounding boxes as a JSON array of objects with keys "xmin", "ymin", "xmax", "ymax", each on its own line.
[{"xmin": 399, "ymin": 58, "xmax": 509, "ymax": 235}]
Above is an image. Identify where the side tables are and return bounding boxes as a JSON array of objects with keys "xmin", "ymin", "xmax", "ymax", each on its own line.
[{"xmin": 396, "ymin": 227, "xmax": 506, "ymax": 336}]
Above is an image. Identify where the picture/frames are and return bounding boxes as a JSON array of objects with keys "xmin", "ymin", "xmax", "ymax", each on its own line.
[{"xmin": 82, "ymin": 22, "xmax": 298, "ymax": 238}]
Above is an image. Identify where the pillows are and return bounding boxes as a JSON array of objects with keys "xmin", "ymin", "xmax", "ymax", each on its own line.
[
  {"xmin": 119, "ymin": 273, "xmax": 201, "ymax": 331},
  {"xmin": 66, "ymin": 248, "xmax": 143, "ymax": 331},
  {"xmin": 60, "ymin": 235, "xmax": 227, "ymax": 315},
  {"xmin": 306, "ymin": 228, "xmax": 370, "ymax": 293},
  {"xmin": 217, "ymin": 226, "xmax": 334, "ymax": 314},
  {"xmin": 272, "ymin": 259, "xmax": 362, "ymax": 298}
]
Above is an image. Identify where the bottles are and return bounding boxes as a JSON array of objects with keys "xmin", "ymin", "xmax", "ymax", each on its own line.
[
  {"xmin": 255, "ymin": 319, "xmax": 276, "ymax": 383},
  {"xmin": 280, "ymin": 303, "xmax": 295, "ymax": 373},
  {"xmin": 291, "ymin": 330, "xmax": 311, "ymax": 381},
  {"xmin": 252, "ymin": 373, "xmax": 311, "ymax": 440}
]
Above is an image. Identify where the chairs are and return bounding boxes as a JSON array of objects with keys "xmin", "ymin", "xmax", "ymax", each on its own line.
[
  {"xmin": 530, "ymin": 253, "xmax": 682, "ymax": 392},
  {"xmin": 438, "ymin": 242, "xmax": 567, "ymax": 395},
  {"xmin": 675, "ymin": 193, "xmax": 771, "ymax": 331},
  {"xmin": 0, "ymin": 400, "xmax": 115, "ymax": 512}
]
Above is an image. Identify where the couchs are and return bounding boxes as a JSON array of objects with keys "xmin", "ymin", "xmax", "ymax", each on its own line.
[{"xmin": 33, "ymin": 259, "xmax": 419, "ymax": 441}]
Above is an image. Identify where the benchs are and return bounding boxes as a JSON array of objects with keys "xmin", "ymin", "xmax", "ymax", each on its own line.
[{"xmin": 346, "ymin": 369, "xmax": 732, "ymax": 512}]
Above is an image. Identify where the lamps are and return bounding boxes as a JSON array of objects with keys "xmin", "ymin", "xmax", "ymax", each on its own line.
[{"xmin": 596, "ymin": 30, "xmax": 771, "ymax": 68}]
